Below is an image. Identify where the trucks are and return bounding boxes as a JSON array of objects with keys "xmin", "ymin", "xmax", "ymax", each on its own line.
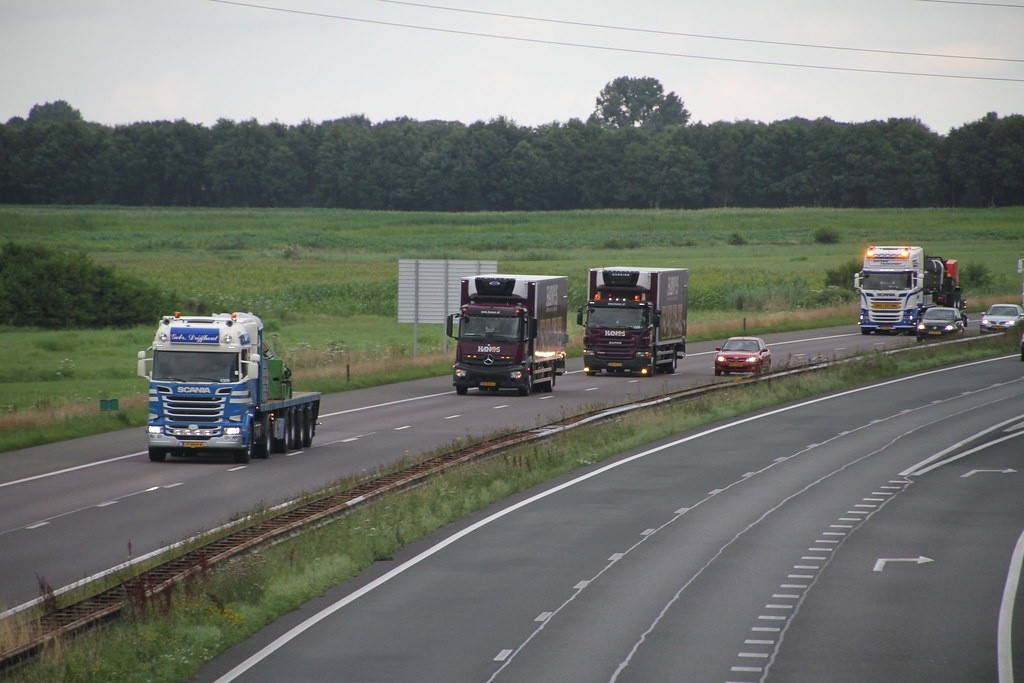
[
  {"xmin": 576, "ymin": 267, "xmax": 689, "ymax": 377},
  {"xmin": 446, "ymin": 274, "xmax": 568, "ymax": 397}
]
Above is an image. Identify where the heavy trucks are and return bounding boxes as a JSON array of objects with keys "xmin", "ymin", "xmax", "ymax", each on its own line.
[
  {"xmin": 854, "ymin": 245, "xmax": 968, "ymax": 336},
  {"xmin": 137, "ymin": 310, "xmax": 323, "ymax": 463}
]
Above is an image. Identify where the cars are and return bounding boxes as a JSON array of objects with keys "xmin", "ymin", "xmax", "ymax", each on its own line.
[
  {"xmin": 979, "ymin": 303, "xmax": 1024, "ymax": 334},
  {"xmin": 916, "ymin": 307, "xmax": 965, "ymax": 343},
  {"xmin": 714, "ymin": 337, "xmax": 772, "ymax": 376}
]
